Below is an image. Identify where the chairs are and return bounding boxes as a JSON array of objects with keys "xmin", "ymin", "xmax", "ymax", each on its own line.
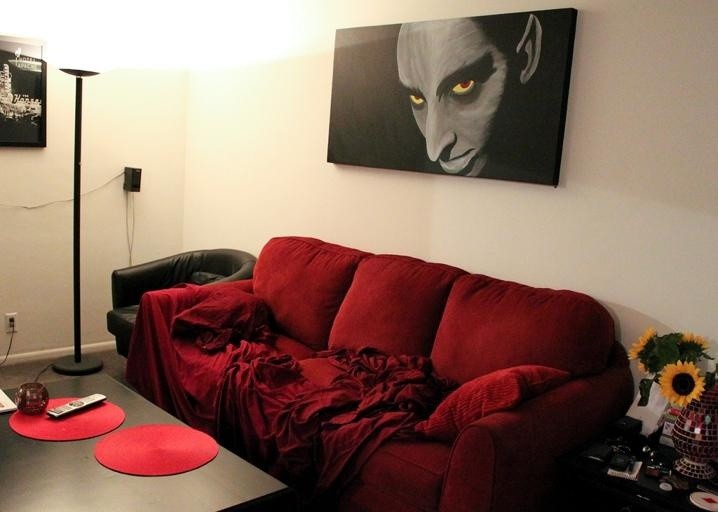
[{"xmin": 107, "ymin": 248, "xmax": 256, "ymax": 359}]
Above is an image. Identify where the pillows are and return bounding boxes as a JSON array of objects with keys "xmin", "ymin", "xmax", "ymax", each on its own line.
[
  {"xmin": 174, "ymin": 286, "xmax": 268, "ymax": 349},
  {"xmin": 413, "ymin": 364, "xmax": 571, "ymax": 442}
]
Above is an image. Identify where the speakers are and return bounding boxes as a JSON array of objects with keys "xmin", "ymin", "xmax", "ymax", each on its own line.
[{"xmin": 123, "ymin": 167, "xmax": 142, "ymax": 192}]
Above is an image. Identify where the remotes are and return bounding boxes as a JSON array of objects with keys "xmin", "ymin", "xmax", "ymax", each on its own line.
[{"xmin": 46, "ymin": 393, "xmax": 107, "ymax": 419}]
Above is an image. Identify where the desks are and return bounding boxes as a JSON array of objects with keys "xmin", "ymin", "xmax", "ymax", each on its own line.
[{"xmin": 554, "ymin": 432, "xmax": 718, "ymax": 512}]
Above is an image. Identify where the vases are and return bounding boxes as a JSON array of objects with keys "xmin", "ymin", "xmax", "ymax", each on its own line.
[{"xmin": 671, "ymin": 381, "xmax": 718, "ymax": 479}]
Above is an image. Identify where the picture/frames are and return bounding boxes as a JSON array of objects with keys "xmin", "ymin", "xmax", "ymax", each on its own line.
[{"xmin": 0, "ymin": 50, "xmax": 46, "ymax": 147}]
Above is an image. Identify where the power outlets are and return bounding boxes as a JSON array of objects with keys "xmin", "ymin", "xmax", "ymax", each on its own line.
[{"xmin": 6, "ymin": 312, "xmax": 19, "ymax": 333}]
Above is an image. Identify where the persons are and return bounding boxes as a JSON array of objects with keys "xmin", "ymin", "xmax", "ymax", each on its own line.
[{"xmin": 397, "ymin": 14, "xmax": 543, "ymax": 178}]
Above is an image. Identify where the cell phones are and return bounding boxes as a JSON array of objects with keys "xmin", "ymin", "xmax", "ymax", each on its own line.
[{"xmin": 609, "ymin": 453, "xmax": 631, "ymax": 472}]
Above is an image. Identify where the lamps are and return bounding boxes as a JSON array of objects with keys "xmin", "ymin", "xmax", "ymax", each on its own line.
[{"xmin": 54, "ymin": 66, "xmax": 104, "ymax": 377}]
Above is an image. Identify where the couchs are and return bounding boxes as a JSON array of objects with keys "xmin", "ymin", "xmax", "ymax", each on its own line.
[{"xmin": 123, "ymin": 236, "xmax": 635, "ymax": 512}]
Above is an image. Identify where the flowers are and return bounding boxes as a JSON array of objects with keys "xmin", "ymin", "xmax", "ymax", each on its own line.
[{"xmin": 628, "ymin": 327, "xmax": 715, "ymax": 407}]
